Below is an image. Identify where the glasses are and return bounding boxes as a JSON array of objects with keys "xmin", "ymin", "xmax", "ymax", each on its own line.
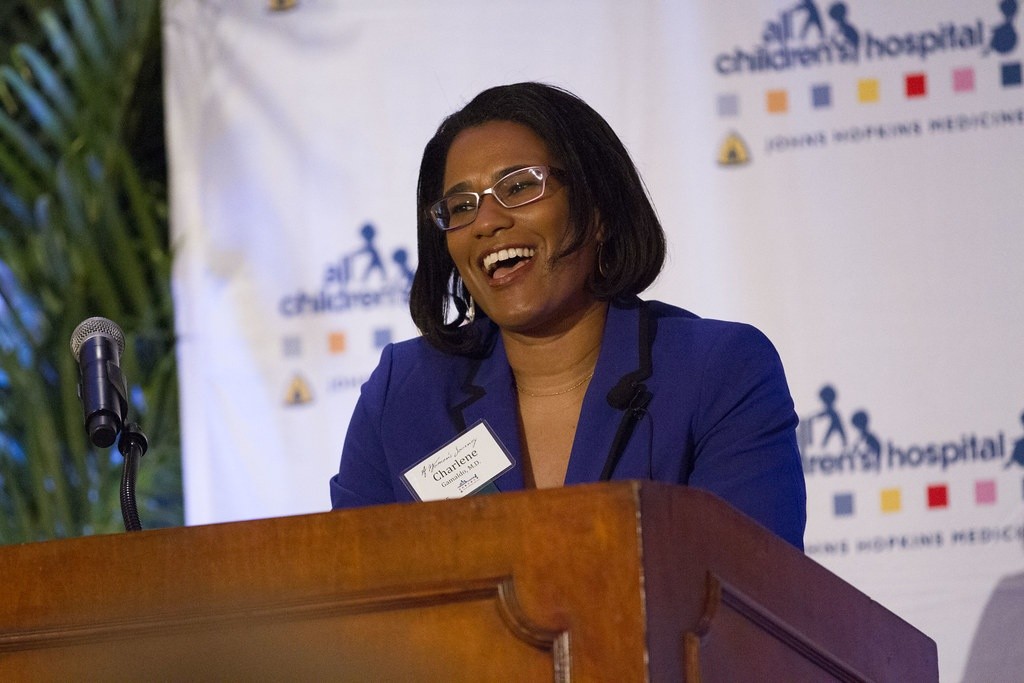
[{"xmin": 431, "ymin": 165, "xmax": 571, "ymax": 231}]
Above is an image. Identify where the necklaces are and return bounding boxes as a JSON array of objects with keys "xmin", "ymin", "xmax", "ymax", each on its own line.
[{"xmin": 514, "ymin": 372, "xmax": 595, "ymax": 397}]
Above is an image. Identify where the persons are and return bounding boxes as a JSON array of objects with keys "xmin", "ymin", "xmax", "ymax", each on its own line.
[{"xmin": 330, "ymin": 84, "xmax": 807, "ymax": 556}]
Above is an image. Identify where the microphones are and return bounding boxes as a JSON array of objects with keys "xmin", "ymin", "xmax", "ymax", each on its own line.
[{"xmin": 70, "ymin": 316, "xmax": 127, "ymax": 448}]
